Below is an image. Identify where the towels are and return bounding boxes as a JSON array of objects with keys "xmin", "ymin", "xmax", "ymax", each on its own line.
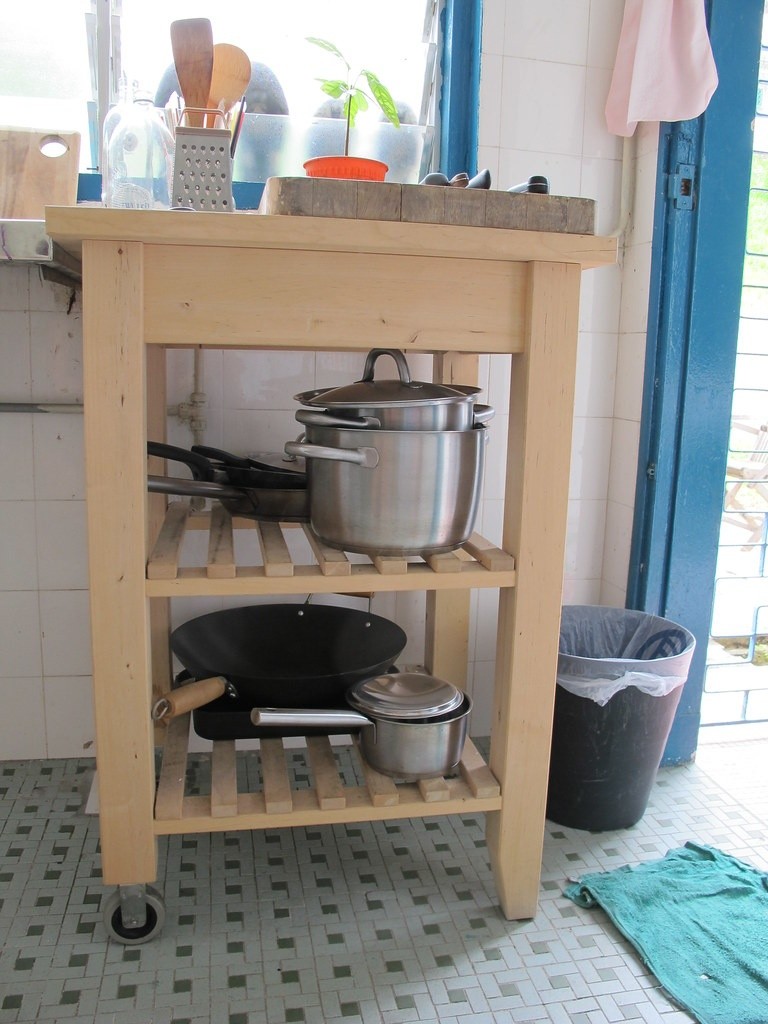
[
  {"xmin": 562, "ymin": 839, "xmax": 768, "ymax": 1024},
  {"xmin": 599, "ymin": 0, "xmax": 721, "ymax": 139}
]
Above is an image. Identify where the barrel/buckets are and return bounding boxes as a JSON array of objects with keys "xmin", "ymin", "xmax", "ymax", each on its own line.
[{"xmin": 285, "ymin": 424, "xmax": 491, "ymax": 557}]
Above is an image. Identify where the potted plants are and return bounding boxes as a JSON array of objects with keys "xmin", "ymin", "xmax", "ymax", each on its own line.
[{"xmin": 303, "ymin": 34, "xmax": 400, "ymax": 182}]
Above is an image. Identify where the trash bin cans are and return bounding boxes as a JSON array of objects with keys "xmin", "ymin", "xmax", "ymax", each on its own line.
[{"xmin": 544, "ymin": 604, "xmax": 698, "ymax": 835}]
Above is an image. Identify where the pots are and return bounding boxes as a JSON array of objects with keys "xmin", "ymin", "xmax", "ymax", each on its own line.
[
  {"xmin": 293, "ymin": 348, "xmax": 495, "ymax": 431},
  {"xmin": 147, "ymin": 441, "xmax": 307, "ymax": 523},
  {"xmin": 251, "ymin": 672, "xmax": 473, "ymax": 781},
  {"xmin": 151, "ymin": 592, "xmax": 408, "ymax": 721}
]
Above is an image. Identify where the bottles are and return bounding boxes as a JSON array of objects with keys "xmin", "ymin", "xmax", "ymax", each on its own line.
[
  {"xmin": 110, "ymin": 83, "xmax": 174, "ymax": 208},
  {"xmin": 101, "ymin": 77, "xmax": 152, "ymax": 206}
]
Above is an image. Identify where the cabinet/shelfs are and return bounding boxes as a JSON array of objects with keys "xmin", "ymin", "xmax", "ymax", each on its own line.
[{"xmin": 44, "ymin": 205, "xmax": 621, "ymax": 918}]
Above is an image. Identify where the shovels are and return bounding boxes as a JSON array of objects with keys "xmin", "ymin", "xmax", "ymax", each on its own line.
[{"xmin": 169, "ymin": 15, "xmax": 252, "ymax": 127}]
